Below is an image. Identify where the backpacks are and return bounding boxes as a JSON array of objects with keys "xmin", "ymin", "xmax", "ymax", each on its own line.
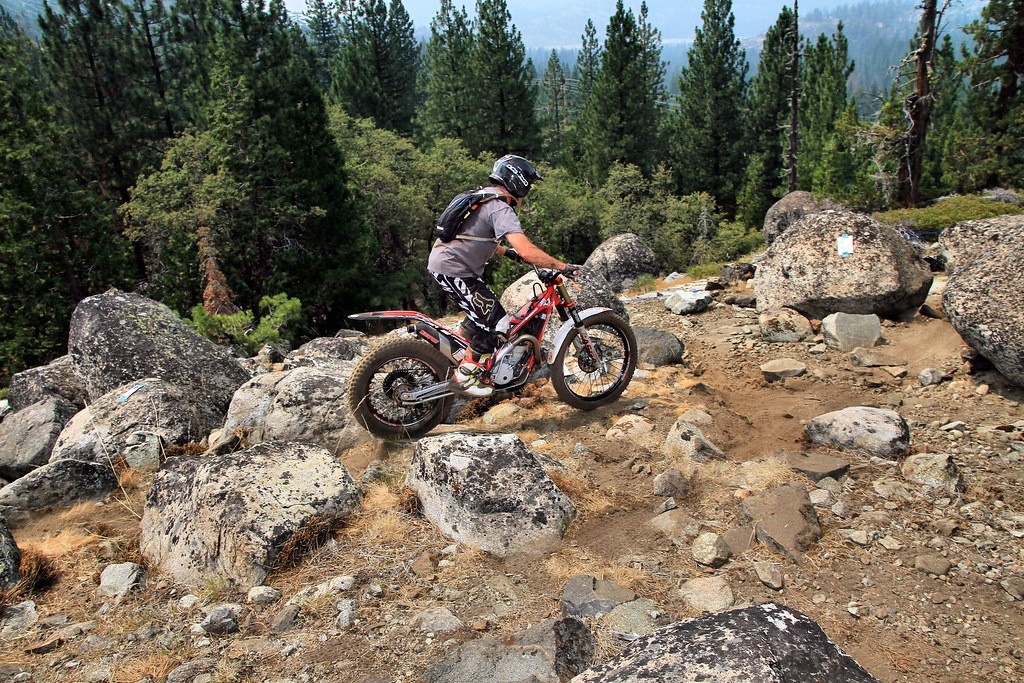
[{"xmin": 436, "ymin": 186, "xmax": 519, "ymax": 243}]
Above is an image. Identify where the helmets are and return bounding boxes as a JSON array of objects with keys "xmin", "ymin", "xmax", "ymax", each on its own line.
[{"xmin": 489, "ymin": 154, "xmax": 545, "ymax": 198}]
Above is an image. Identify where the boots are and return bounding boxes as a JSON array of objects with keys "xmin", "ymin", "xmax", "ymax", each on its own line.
[{"xmin": 447, "ymin": 359, "xmax": 495, "ymax": 398}]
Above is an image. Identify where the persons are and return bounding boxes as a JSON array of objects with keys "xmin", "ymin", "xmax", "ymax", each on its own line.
[{"xmin": 427, "ymin": 154, "xmax": 579, "ymax": 398}]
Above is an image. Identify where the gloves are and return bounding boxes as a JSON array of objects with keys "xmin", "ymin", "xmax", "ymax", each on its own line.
[
  {"xmin": 561, "ymin": 265, "xmax": 580, "ymax": 278},
  {"xmin": 504, "ymin": 248, "xmax": 523, "ymax": 263}
]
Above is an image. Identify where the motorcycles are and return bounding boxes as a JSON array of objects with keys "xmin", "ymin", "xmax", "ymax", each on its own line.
[{"xmin": 346, "ymin": 261, "xmax": 638, "ymax": 442}]
